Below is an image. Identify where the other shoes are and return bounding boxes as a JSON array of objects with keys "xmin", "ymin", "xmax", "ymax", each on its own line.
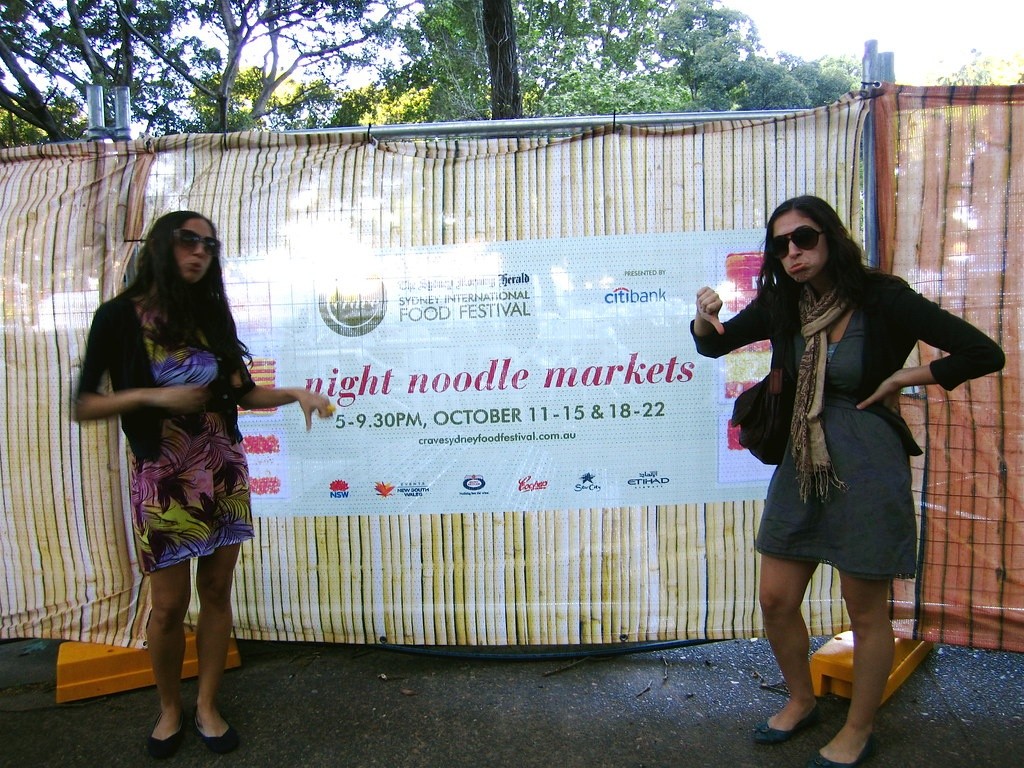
[
  {"xmin": 192, "ymin": 706, "xmax": 239, "ymax": 752},
  {"xmin": 806, "ymin": 732, "xmax": 873, "ymax": 768},
  {"xmin": 149, "ymin": 710, "xmax": 185, "ymax": 759},
  {"xmin": 755, "ymin": 704, "xmax": 820, "ymax": 744}
]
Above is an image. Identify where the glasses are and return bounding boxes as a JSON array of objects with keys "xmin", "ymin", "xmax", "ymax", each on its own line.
[
  {"xmin": 768, "ymin": 227, "xmax": 825, "ymax": 260},
  {"xmin": 173, "ymin": 229, "xmax": 221, "ymax": 256}
]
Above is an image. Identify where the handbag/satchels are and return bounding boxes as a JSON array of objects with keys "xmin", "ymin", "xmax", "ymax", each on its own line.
[{"xmin": 732, "ymin": 288, "xmax": 793, "ymax": 465}]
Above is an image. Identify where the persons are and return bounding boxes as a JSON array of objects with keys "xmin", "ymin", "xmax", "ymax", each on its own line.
[
  {"xmin": 689, "ymin": 194, "xmax": 1006, "ymax": 768},
  {"xmin": 71, "ymin": 210, "xmax": 333, "ymax": 756}
]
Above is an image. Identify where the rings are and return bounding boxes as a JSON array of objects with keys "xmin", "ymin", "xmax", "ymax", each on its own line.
[{"xmin": 327, "ymin": 404, "xmax": 337, "ymax": 413}]
